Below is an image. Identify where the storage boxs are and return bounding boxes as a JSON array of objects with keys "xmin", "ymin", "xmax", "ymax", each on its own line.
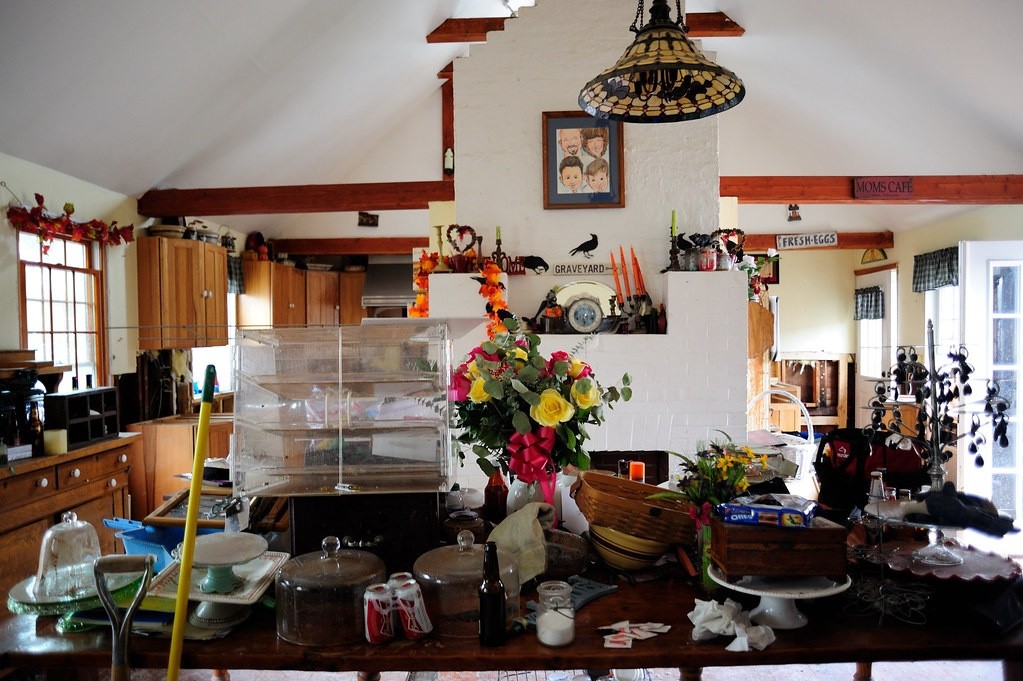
[
  {"xmin": 7, "ymin": 445, "xmax": 33, "ymax": 462},
  {"xmin": 708, "ymin": 513, "xmax": 848, "ymax": 578}
]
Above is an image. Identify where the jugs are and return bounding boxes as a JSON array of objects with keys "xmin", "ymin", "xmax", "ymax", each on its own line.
[{"xmin": 717, "ymin": 252, "xmax": 737, "ymax": 270}]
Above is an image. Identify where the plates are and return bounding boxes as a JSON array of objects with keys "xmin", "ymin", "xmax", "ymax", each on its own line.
[
  {"xmin": 8, "ymin": 562, "xmax": 143, "ymax": 603},
  {"xmin": 567, "ymin": 298, "xmax": 603, "ymax": 333}
]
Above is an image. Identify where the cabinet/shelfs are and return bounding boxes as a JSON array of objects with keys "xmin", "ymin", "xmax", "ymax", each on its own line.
[
  {"xmin": 46, "ymin": 384, "xmax": 121, "ymax": 451},
  {"xmin": 0, "ymin": 432, "xmax": 140, "ymax": 616},
  {"xmin": 747, "ymin": 302, "xmax": 801, "ymax": 434},
  {"xmin": 236, "ymin": 260, "xmax": 367, "ymax": 331},
  {"xmin": 137, "ymin": 236, "xmax": 229, "ymax": 350},
  {"xmin": 127, "ymin": 411, "xmax": 236, "ymax": 520}
]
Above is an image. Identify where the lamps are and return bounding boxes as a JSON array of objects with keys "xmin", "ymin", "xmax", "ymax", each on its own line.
[{"xmin": 578, "ymin": 1, "xmax": 746, "ymax": 124}]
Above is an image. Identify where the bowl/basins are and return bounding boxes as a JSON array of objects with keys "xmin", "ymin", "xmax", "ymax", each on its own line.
[
  {"xmin": 589, "ymin": 523, "xmax": 671, "ymax": 574},
  {"xmin": 150, "ymin": 225, "xmax": 187, "ymax": 238},
  {"xmin": 198, "ymin": 231, "xmax": 219, "ymax": 244}
]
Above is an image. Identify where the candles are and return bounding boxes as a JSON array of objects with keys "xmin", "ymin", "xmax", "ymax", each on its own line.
[
  {"xmin": 672, "ymin": 210, "xmax": 677, "ymax": 236},
  {"xmin": 630, "ymin": 247, "xmax": 646, "ymax": 296},
  {"xmin": 610, "ymin": 252, "xmax": 623, "ymax": 305},
  {"xmin": 496, "ymin": 226, "xmax": 500, "ymax": 240},
  {"xmin": 619, "ymin": 244, "xmax": 630, "ymax": 298}
]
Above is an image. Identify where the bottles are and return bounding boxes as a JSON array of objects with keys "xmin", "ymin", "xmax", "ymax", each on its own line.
[
  {"xmin": 485, "ymin": 466, "xmax": 509, "ymax": 522},
  {"xmin": 6, "ymin": 407, "xmax": 21, "ymax": 447},
  {"xmin": 918, "ymin": 485, "xmax": 931, "ymax": 495},
  {"xmin": 685, "ymin": 249, "xmax": 718, "ymax": 271},
  {"xmin": 478, "ymin": 541, "xmax": 507, "ymax": 647},
  {"xmin": 28, "ymin": 401, "xmax": 44, "ymax": 457},
  {"xmin": 869, "ymin": 471, "xmax": 911, "ymax": 502},
  {"xmin": 73, "ymin": 375, "xmax": 92, "ymax": 390},
  {"xmin": 537, "ymin": 580, "xmax": 576, "ymax": 646},
  {"xmin": 618, "ymin": 460, "xmax": 645, "ymax": 483},
  {"xmin": 444, "ymin": 483, "xmax": 484, "ymax": 542}
]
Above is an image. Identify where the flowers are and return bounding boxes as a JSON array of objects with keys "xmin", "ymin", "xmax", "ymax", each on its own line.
[
  {"xmin": 408, "ymin": 247, "xmax": 507, "ymax": 342},
  {"xmin": 646, "ymin": 429, "xmax": 768, "ymax": 530},
  {"xmin": 448, "ymin": 312, "xmax": 633, "ymax": 483},
  {"xmin": 7, "ymin": 192, "xmax": 136, "ymax": 256},
  {"xmin": 733, "ymin": 248, "xmax": 782, "ymax": 306}
]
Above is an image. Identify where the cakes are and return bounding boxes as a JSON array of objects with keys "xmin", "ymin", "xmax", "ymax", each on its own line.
[{"xmin": 286, "ymin": 580, "xmax": 367, "ymax": 646}]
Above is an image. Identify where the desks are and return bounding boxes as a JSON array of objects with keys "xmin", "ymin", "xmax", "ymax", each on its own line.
[{"xmin": 0, "ymin": 569, "xmax": 1023, "ymax": 681}]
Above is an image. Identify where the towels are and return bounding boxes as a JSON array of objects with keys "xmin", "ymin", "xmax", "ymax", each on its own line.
[{"xmin": 486, "ymin": 502, "xmax": 556, "ymax": 593}]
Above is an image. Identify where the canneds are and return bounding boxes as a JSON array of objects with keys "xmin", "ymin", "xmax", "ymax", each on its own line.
[{"xmin": 363, "ymin": 572, "xmax": 434, "ymax": 645}]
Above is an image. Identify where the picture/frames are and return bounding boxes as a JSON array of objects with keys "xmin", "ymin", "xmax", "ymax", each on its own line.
[
  {"xmin": 748, "ymin": 254, "xmax": 779, "ymax": 284},
  {"xmin": 541, "ymin": 111, "xmax": 626, "ymax": 210}
]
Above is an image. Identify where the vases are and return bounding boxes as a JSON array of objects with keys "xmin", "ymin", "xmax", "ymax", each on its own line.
[{"xmin": 507, "ymin": 478, "xmax": 544, "ymax": 517}]
